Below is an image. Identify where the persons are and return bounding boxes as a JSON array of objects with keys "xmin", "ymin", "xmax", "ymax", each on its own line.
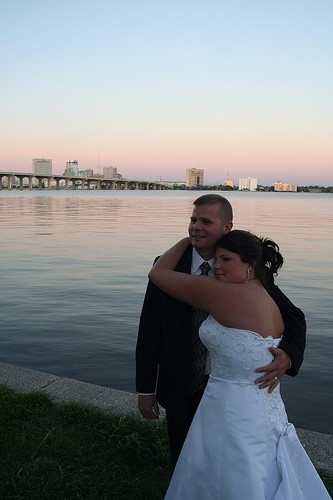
[
  {"xmin": 135, "ymin": 194, "xmax": 308, "ymax": 471},
  {"xmin": 147, "ymin": 229, "xmax": 333, "ymax": 500}
]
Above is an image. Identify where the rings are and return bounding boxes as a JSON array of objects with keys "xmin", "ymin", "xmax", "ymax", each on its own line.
[{"xmin": 274, "ymin": 377, "xmax": 278, "ymax": 381}]
